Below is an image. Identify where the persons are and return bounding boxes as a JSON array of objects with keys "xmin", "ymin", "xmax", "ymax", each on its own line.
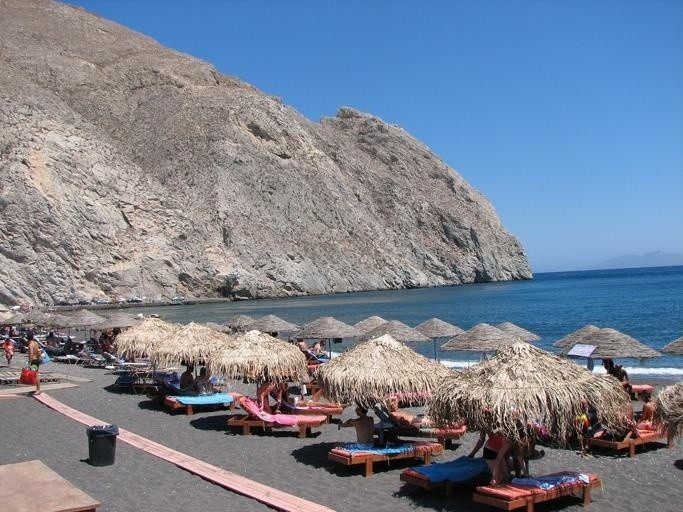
[
  {"xmin": 339, "ymin": 399, "xmax": 466, "ymax": 450},
  {"xmin": 180, "ymin": 364, "xmax": 222, "ymax": 395},
  {"xmin": 2, "ymin": 325, "xmax": 120, "ymax": 395},
  {"xmin": 257, "ymin": 374, "xmax": 345, "ymax": 414},
  {"xmin": 468, "ymin": 414, "xmax": 545, "ymax": 484},
  {"xmin": 272, "ymin": 332, "xmax": 329, "ymax": 361},
  {"xmin": 575, "ymin": 358, "xmax": 656, "ymax": 458}
]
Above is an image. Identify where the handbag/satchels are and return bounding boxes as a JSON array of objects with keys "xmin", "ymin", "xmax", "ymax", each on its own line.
[
  {"xmin": 379, "ymin": 427, "xmax": 400, "ymax": 447},
  {"xmin": 20, "ymin": 366, "xmax": 37, "ymax": 385},
  {"xmin": 38, "ymin": 344, "xmax": 50, "ymax": 364}
]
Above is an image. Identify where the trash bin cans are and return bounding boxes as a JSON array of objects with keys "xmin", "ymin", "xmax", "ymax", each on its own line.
[{"xmin": 86, "ymin": 424, "xmax": 119, "ymax": 467}]
